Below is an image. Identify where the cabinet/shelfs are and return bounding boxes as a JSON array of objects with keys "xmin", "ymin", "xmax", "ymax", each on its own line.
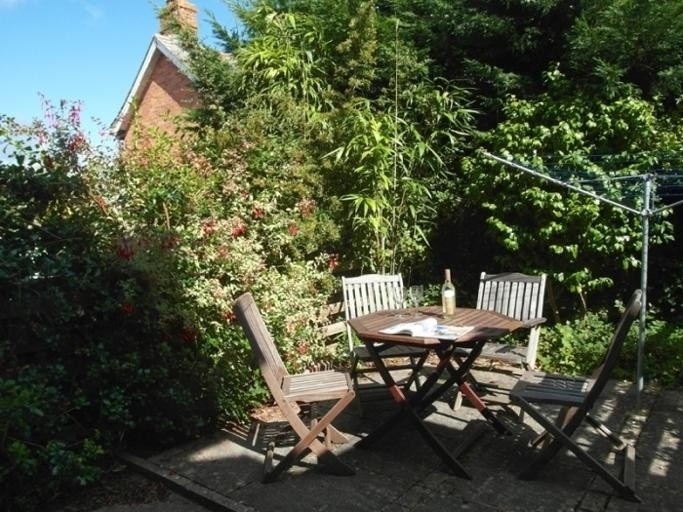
[{"xmin": 441, "ymin": 269, "xmax": 457, "ymax": 316}]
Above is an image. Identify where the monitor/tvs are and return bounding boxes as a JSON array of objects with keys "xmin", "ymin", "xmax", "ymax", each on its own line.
[{"xmin": 381, "ymin": 317, "xmax": 476, "ymax": 342}]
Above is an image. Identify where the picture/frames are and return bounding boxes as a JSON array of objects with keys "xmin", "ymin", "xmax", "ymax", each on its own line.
[{"xmin": 346, "ymin": 304, "xmax": 523, "ymax": 480}]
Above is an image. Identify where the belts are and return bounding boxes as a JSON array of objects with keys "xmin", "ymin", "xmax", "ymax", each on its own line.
[{"xmin": 394, "ymin": 286, "xmax": 424, "ymax": 320}]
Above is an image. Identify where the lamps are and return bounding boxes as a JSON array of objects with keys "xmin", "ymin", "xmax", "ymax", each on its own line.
[
  {"xmin": 510, "ymin": 290, "xmax": 643, "ymax": 501},
  {"xmin": 453, "ymin": 272, "xmax": 547, "ymax": 421},
  {"xmin": 235, "ymin": 292, "xmax": 356, "ymax": 482},
  {"xmin": 341, "ymin": 272, "xmax": 423, "ymax": 416}
]
